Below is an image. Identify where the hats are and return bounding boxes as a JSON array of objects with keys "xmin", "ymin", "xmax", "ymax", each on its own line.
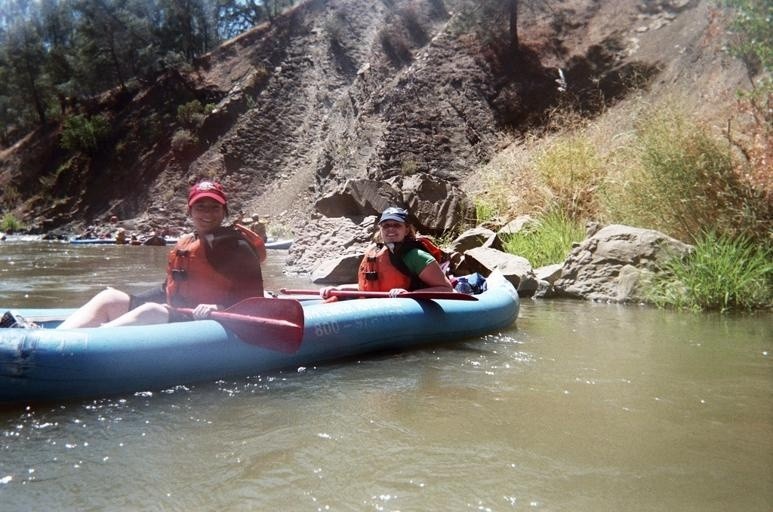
[
  {"xmin": 378, "ymin": 206, "xmax": 409, "ymax": 224},
  {"xmin": 188, "ymin": 181, "xmax": 226, "ymax": 207}
]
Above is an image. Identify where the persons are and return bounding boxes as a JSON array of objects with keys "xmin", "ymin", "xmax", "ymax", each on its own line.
[
  {"xmin": 0, "ymin": 179, "xmax": 265, "ymax": 334},
  {"xmin": 73, "ymin": 209, "xmax": 268, "ymax": 246},
  {"xmin": 319, "ymin": 205, "xmax": 454, "ymax": 301}
]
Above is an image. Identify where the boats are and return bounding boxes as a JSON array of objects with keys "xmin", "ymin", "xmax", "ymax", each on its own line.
[{"xmin": 0, "ymin": 266, "xmax": 521, "ymax": 406}]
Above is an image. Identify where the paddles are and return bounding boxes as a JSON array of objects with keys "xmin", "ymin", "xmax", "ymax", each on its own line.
[
  {"xmin": 169, "ymin": 298, "xmax": 303, "ymax": 353},
  {"xmin": 282, "ymin": 287, "xmax": 480, "ymax": 304}
]
